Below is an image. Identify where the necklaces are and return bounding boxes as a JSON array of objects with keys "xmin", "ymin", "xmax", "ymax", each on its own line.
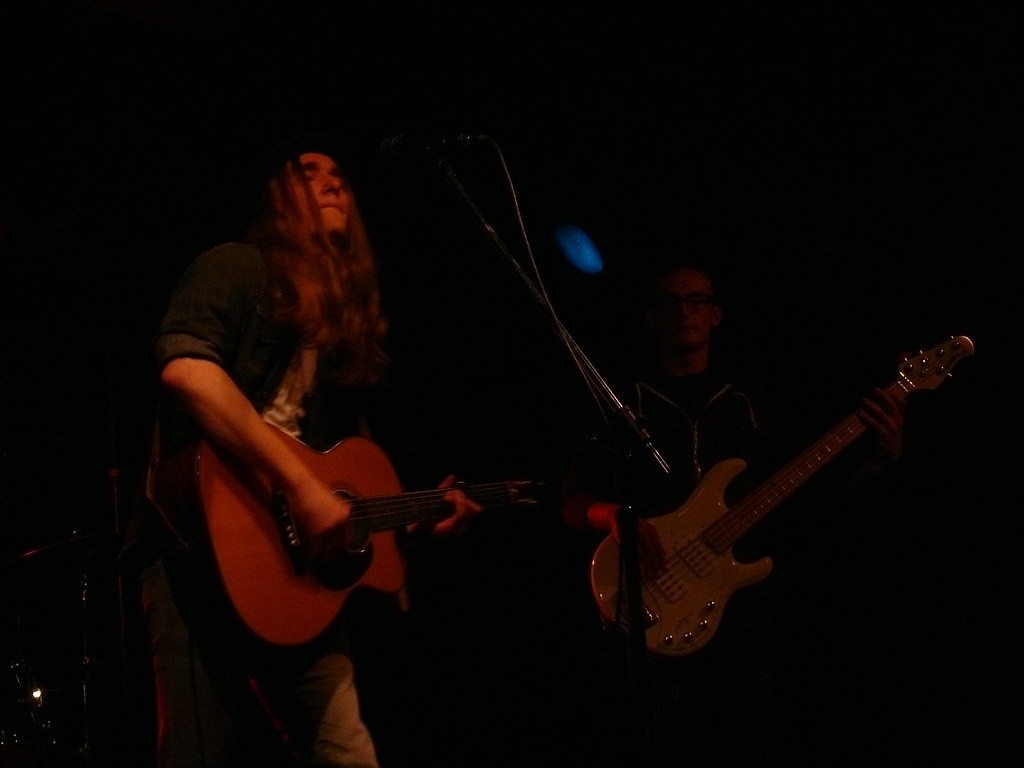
[{"xmin": 282, "ymin": 348, "xmax": 322, "ymax": 408}]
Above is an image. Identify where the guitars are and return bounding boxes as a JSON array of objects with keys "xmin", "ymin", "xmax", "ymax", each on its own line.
[
  {"xmin": 581, "ymin": 335, "xmax": 974, "ymax": 658},
  {"xmin": 162, "ymin": 423, "xmax": 547, "ymax": 671}
]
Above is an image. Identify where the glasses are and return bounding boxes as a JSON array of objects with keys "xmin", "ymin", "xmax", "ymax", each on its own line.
[{"xmin": 656, "ymin": 291, "xmax": 716, "ymax": 312}]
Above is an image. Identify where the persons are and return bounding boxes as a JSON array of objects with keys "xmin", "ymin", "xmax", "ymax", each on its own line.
[
  {"xmin": 556, "ymin": 259, "xmax": 909, "ymax": 768},
  {"xmin": 132, "ymin": 132, "xmax": 491, "ymax": 768}
]
